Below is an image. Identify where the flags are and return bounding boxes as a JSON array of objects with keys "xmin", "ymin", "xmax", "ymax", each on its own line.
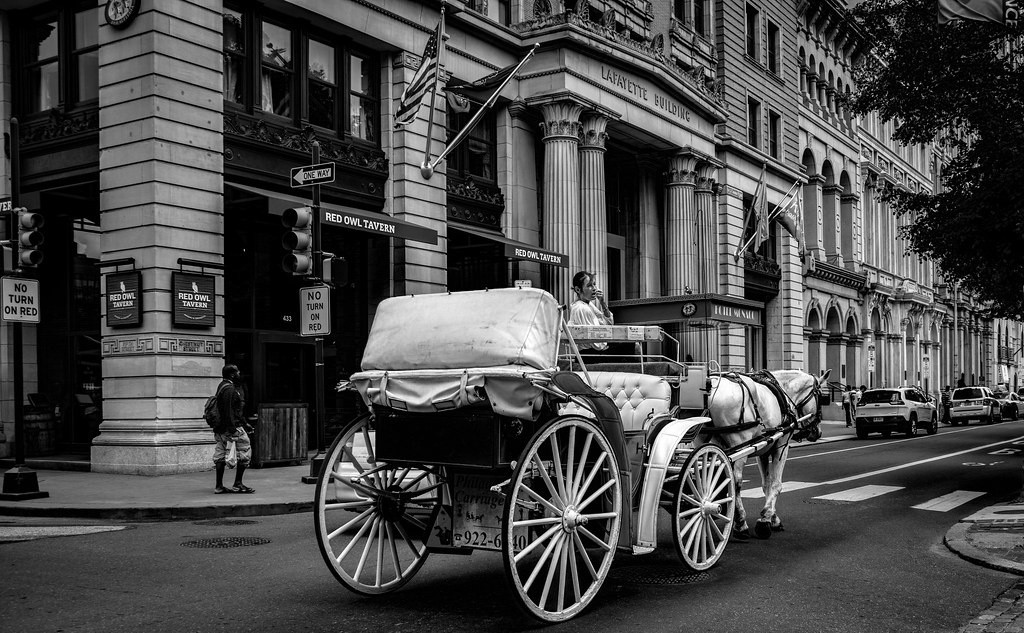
[
  {"xmin": 442, "ymin": 60, "xmax": 519, "ymax": 110},
  {"xmin": 753, "ymin": 173, "xmax": 769, "ymax": 253},
  {"xmin": 394, "ymin": 21, "xmax": 445, "ymax": 128},
  {"xmin": 775, "ymin": 189, "xmax": 806, "ymax": 265}
]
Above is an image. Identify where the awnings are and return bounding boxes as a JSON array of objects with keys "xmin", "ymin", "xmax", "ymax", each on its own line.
[
  {"xmin": 224, "ymin": 181, "xmax": 438, "ymax": 245},
  {"xmin": 450, "ymin": 223, "xmax": 569, "ymax": 268}
]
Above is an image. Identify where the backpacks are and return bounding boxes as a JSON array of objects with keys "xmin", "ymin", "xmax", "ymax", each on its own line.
[{"xmin": 204, "ymin": 382, "xmax": 231, "ymax": 435}]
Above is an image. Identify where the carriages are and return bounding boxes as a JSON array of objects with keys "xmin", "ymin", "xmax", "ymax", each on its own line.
[{"xmin": 314, "ymin": 283, "xmax": 833, "ymax": 627}]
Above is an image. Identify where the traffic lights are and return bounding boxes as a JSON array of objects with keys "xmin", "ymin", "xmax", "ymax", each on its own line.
[
  {"xmin": 279, "ymin": 206, "xmax": 314, "ymax": 276},
  {"xmin": 15, "ymin": 210, "xmax": 45, "ymax": 271}
]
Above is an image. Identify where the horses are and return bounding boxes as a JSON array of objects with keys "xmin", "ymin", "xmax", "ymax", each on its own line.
[{"xmin": 707, "ymin": 369, "xmax": 832, "ymax": 540}]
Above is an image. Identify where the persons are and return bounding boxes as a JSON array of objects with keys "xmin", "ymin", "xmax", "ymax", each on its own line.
[
  {"xmin": 841, "ymin": 385, "xmax": 867, "ymax": 428},
  {"xmin": 941, "ymin": 386, "xmax": 952, "ymax": 424},
  {"xmin": 213, "ymin": 364, "xmax": 255, "ymax": 494},
  {"xmin": 567, "ymin": 271, "xmax": 635, "ymax": 362}
]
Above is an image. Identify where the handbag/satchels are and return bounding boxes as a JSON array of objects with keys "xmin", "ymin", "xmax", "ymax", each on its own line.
[{"xmin": 225, "ymin": 441, "xmax": 237, "ymax": 469}]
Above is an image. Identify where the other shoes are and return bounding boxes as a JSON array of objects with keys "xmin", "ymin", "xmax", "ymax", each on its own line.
[
  {"xmin": 232, "ymin": 483, "xmax": 256, "ymax": 493},
  {"xmin": 214, "ymin": 486, "xmax": 237, "ymax": 495}
]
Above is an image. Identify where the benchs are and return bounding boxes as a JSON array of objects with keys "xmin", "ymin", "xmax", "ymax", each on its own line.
[{"xmin": 556, "ymin": 370, "xmax": 673, "ymax": 430}]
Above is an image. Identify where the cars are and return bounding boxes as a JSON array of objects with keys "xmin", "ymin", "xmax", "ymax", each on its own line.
[
  {"xmin": 992, "ymin": 391, "xmax": 1024, "ymax": 421},
  {"xmin": 1017, "ymin": 387, "xmax": 1024, "ymax": 397}
]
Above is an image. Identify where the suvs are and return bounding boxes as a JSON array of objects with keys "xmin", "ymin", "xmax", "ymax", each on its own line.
[
  {"xmin": 949, "ymin": 386, "xmax": 1002, "ymax": 427},
  {"xmin": 854, "ymin": 384, "xmax": 938, "ymax": 438}
]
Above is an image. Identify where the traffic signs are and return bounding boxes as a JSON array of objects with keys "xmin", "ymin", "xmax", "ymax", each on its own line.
[{"xmin": 290, "ymin": 161, "xmax": 336, "ymax": 188}]
